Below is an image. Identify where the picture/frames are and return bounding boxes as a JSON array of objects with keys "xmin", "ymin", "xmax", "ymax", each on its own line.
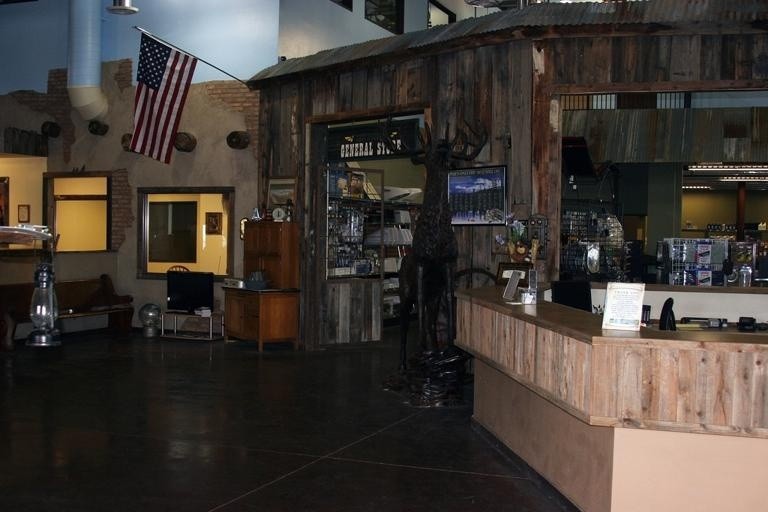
[
  {"xmin": 18, "ymin": 205, "xmax": 30, "ymax": 222},
  {"xmin": 206, "ymin": 213, "xmax": 222, "ymax": 234},
  {"xmin": 447, "ymin": 165, "xmax": 507, "ymax": 226}
]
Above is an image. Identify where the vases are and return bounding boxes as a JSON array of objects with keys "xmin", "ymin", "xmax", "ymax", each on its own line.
[{"xmin": 508, "ymin": 239, "xmax": 539, "ymax": 263}]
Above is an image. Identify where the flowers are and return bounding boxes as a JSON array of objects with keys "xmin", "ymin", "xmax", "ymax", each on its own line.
[{"xmin": 496, "ymin": 210, "xmax": 526, "ymax": 246}]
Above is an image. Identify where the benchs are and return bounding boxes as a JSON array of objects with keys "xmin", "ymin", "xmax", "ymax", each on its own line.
[{"xmin": 0, "ymin": 274, "xmax": 134, "ymax": 352}]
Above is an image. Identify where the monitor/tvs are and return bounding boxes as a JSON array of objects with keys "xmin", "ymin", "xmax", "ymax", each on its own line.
[
  {"xmin": 496, "ymin": 261, "xmax": 533, "ymax": 289},
  {"xmin": 550, "ymin": 279, "xmax": 593, "ymax": 313},
  {"xmin": 165, "ymin": 271, "xmax": 215, "ymax": 315}
]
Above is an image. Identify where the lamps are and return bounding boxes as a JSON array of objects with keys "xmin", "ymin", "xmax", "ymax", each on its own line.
[{"xmin": 26, "ymin": 234, "xmax": 61, "ymax": 346}]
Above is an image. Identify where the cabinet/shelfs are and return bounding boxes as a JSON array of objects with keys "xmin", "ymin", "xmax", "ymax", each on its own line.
[
  {"xmin": 221, "ymin": 221, "xmax": 303, "ymax": 353},
  {"xmin": 160, "ymin": 310, "xmax": 225, "ymax": 341},
  {"xmin": 324, "ymin": 197, "xmax": 423, "ymax": 328}
]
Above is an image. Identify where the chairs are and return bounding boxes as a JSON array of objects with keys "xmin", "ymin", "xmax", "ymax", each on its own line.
[{"xmin": 167, "ymin": 265, "xmax": 190, "ymax": 272}]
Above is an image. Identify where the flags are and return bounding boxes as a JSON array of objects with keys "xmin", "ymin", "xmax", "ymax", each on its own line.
[{"xmin": 128, "ymin": 31, "xmax": 198, "ymax": 165}]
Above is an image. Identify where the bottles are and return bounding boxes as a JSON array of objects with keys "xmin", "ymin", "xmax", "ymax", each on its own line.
[
  {"xmin": 738, "ymin": 263, "xmax": 752, "ymax": 287},
  {"xmin": 287, "ymin": 198, "xmax": 294, "ymax": 221}
]
